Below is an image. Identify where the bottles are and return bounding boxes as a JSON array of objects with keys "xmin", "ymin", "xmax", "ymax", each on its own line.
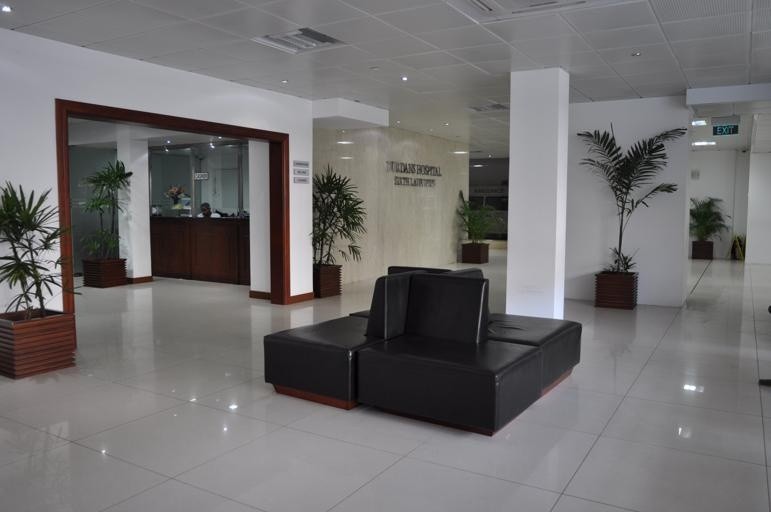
[
  {"xmin": 152, "ymin": 205, "xmax": 157, "ymax": 216},
  {"xmin": 157, "ymin": 205, "xmax": 162, "ymax": 216}
]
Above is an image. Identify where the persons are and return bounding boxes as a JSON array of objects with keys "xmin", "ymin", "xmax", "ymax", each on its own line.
[{"xmin": 196, "ymin": 202, "xmax": 221, "ymax": 218}]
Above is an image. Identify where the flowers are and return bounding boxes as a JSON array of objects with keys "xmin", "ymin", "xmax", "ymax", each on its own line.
[{"xmin": 161, "ymin": 183, "xmax": 191, "ymax": 209}]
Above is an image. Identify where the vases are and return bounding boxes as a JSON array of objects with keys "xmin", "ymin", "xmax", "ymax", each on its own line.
[{"xmin": 170, "ymin": 202, "xmax": 183, "ymax": 209}]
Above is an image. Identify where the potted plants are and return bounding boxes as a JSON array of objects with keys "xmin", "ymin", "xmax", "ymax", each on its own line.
[
  {"xmin": 455, "ymin": 190, "xmax": 504, "ymax": 264},
  {"xmin": 576, "ymin": 122, "xmax": 687, "ymax": 310},
  {"xmin": 312, "ymin": 163, "xmax": 368, "ymax": 299},
  {"xmin": 689, "ymin": 196, "xmax": 732, "ymax": 260},
  {"xmin": 82, "ymin": 160, "xmax": 133, "ymax": 289},
  {"xmin": 0, "ymin": 178, "xmax": 83, "ymax": 381}
]
